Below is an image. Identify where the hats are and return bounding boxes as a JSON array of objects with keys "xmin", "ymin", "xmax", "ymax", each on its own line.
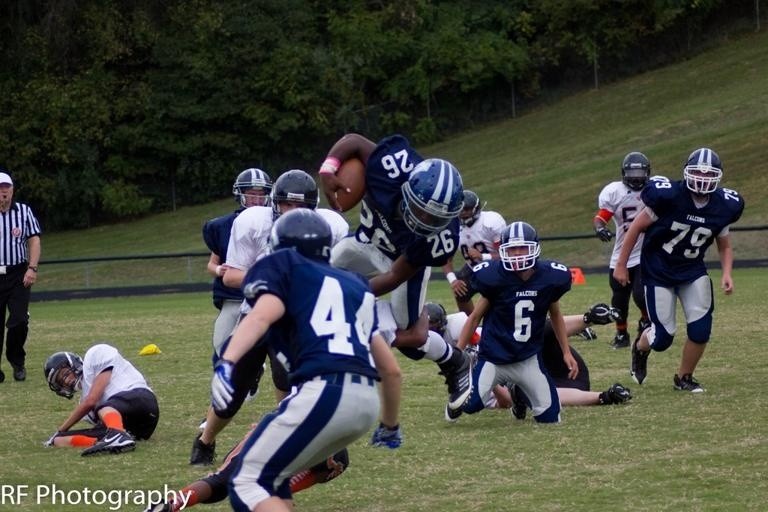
[{"xmin": 0, "ymin": 172, "xmax": 13, "ymax": 185}]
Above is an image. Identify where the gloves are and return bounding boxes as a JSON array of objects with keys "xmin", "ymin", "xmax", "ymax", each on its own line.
[
  {"xmin": 369, "ymin": 421, "xmax": 403, "ymax": 448},
  {"xmin": 210, "ymin": 361, "xmax": 236, "ymax": 411},
  {"xmin": 595, "ymin": 226, "xmax": 614, "ymax": 243}
]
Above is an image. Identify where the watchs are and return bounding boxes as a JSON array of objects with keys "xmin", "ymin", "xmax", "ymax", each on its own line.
[{"xmin": 28, "ymin": 265, "xmax": 38, "ymax": 272}]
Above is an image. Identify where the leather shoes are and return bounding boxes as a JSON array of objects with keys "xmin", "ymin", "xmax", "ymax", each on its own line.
[{"xmin": 10, "ymin": 360, "xmax": 26, "ymax": 381}]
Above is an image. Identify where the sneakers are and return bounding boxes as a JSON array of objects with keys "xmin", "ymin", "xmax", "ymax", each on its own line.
[
  {"xmin": 81, "ymin": 427, "xmax": 136, "ymax": 457},
  {"xmin": 576, "ymin": 302, "xmax": 707, "ymax": 405},
  {"xmin": 438, "ymin": 346, "xmax": 528, "ymax": 423},
  {"xmin": 189, "ymin": 434, "xmax": 216, "ymax": 467}
]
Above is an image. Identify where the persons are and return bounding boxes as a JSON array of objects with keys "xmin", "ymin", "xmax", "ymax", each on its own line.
[
  {"xmin": 0, "ymin": 172, "xmax": 42, "ymax": 382},
  {"xmin": 613, "ymin": 147, "xmax": 745, "ymax": 394},
  {"xmin": 592, "ymin": 152, "xmax": 670, "ymax": 348},
  {"xmin": 143, "ymin": 133, "xmax": 633, "ymax": 512},
  {"xmin": 43, "ymin": 343, "xmax": 159, "ymax": 456}
]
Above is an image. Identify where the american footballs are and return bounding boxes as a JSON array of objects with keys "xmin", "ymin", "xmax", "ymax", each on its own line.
[{"xmin": 334, "ymin": 157, "xmax": 367, "ymax": 211}]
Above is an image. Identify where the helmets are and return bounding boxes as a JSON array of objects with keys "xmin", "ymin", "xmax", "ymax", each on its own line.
[
  {"xmin": 232, "ymin": 167, "xmax": 331, "ymax": 262},
  {"xmin": 401, "ymin": 157, "xmax": 542, "ymax": 334},
  {"xmin": 44, "ymin": 352, "xmax": 83, "ymax": 400},
  {"xmin": 683, "ymin": 148, "xmax": 724, "ymax": 195},
  {"xmin": 622, "ymin": 152, "xmax": 651, "ymax": 191}
]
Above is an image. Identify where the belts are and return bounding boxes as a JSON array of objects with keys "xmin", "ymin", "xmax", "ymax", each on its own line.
[{"xmin": 0, "ymin": 263, "xmax": 26, "ymax": 275}]
